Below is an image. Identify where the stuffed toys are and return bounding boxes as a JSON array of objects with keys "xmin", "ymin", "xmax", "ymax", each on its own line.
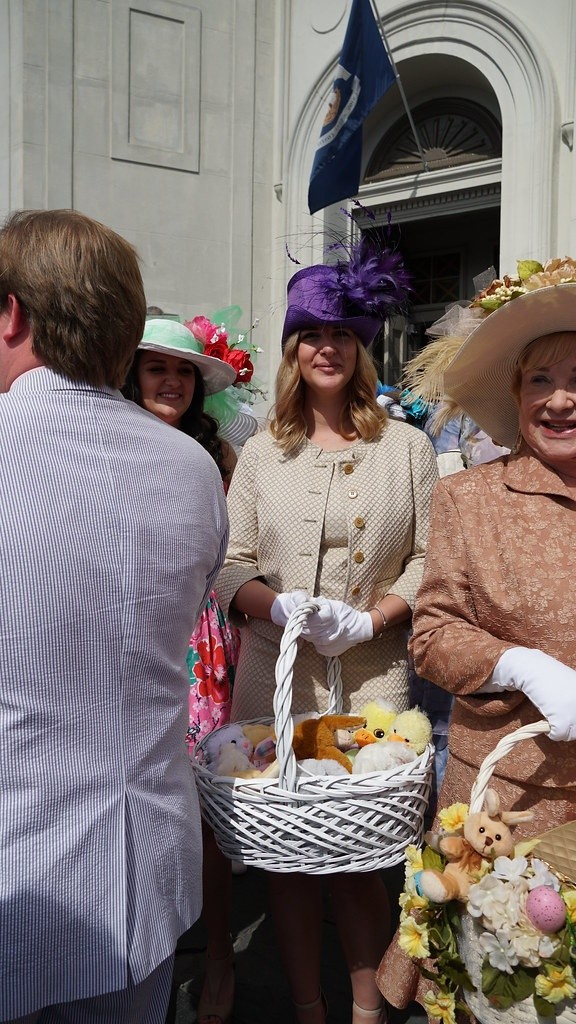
[
  {"xmin": 206, "ymin": 697, "xmax": 432, "ymax": 778},
  {"xmin": 423, "ymin": 789, "xmax": 533, "ymax": 903}
]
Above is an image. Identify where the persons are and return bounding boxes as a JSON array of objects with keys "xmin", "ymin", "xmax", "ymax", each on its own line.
[
  {"xmin": 377, "ymin": 382, "xmax": 511, "ymax": 479},
  {"xmin": 128, "ymin": 315, "xmax": 257, "ymax": 1023},
  {"xmin": 0, "ymin": 211, "xmax": 231, "ymax": 1023},
  {"xmin": 375, "ymin": 257, "xmax": 576, "ymax": 1024},
  {"xmin": 210, "ymin": 265, "xmax": 443, "ymax": 1023}
]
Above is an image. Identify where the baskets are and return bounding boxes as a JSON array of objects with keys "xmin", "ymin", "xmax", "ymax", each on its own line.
[
  {"xmin": 188, "ymin": 603, "xmax": 436, "ymax": 876},
  {"xmin": 456, "ymin": 720, "xmax": 574, "ymax": 1024}
]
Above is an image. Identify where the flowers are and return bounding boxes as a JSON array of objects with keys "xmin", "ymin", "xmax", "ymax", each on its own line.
[
  {"xmin": 465, "ymin": 255, "xmax": 576, "ymax": 312},
  {"xmin": 185, "ymin": 315, "xmax": 268, "ymax": 406},
  {"xmin": 398, "ymin": 802, "xmax": 576, "ymax": 1024}
]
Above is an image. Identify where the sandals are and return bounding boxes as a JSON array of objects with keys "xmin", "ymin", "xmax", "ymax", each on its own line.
[
  {"xmin": 292, "ymin": 985, "xmax": 330, "ymax": 1024},
  {"xmin": 352, "ymin": 991, "xmax": 391, "ymax": 1024},
  {"xmin": 198, "ymin": 935, "xmax": 235, "ymax": 1024}
]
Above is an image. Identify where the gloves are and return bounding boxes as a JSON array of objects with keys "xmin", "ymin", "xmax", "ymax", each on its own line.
[
  {"xmin": 308, "ymin": 596, "xmax": 373, "ymax": 657},
  {"xmin": 272, "ymin": 591, "xmax": 337, "ymax": 643},
  {"xmin": 478, "ymin": 646, "xmax": 576, "ymax": 742}
]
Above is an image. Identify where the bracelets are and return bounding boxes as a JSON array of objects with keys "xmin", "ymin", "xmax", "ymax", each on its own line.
[{"xmin": 368, "ymin": 606, "xmax": 386, "ymax": 640}]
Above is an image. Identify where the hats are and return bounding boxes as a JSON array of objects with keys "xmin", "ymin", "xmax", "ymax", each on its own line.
[
  {"xmin": 204, "ymin": 390, "xmax": 258, "ymax": 453},
  {"xmin": 137, "ymin": 307, "xmax": 265, "ymax": 397},
  {"xmin": 281, "ymin": 198, "xmax": 412, "ymax": 348},
  {"xmin": 400, "ymin": 256, "xmax": 575, "ymax": 448}
]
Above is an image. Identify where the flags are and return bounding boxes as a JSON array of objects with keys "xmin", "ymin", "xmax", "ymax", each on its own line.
[{"xmin": 308, "ymin": 0, "xmax": 419, "ymax": 216}]
